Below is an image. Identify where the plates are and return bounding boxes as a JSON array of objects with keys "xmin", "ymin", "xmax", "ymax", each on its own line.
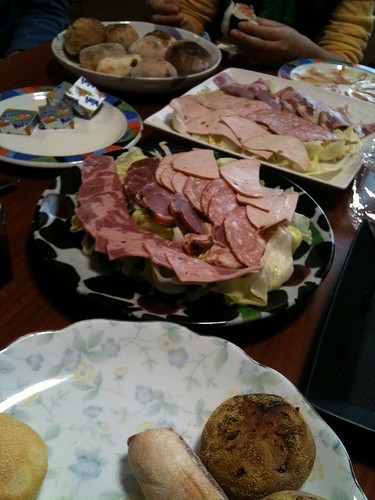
[
  {"xmin": 0, "ymin": 85, "xmax": 143, "ymax": 167},
  {"xmin": 278, "ymin": 58, "xmax": 374, "ymax": 104},
  {"xmin": 0, "ymin": 316, "xmax": 369, "ymax": 500},
  {"xmin": 143, "ymin": 66, "xmax": 375, "ymax": 190},
  {"xmin": 26, "ymin": 140, "xmax": 336, "ymax": 328}
]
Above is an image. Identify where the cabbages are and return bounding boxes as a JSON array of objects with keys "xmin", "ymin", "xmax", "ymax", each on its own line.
[
  {"xmin": 197, "ymin": 81, "xmax": 361, "ymax": 171},
  {"xmin": 69, "ymin": 151, "xmax": 312, "ymax": 307}
]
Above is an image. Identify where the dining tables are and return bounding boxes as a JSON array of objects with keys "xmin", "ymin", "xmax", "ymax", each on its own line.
[{"xmin": 0, "ymin": 45, "xmax": 364, "ymax": 407}]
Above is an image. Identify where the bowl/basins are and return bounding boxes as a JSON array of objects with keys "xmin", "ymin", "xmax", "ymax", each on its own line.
[{"xmin": 51, "ymin": 20, "xmax": 223, "ymax": 88}]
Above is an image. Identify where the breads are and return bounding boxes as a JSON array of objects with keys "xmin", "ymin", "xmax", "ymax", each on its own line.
[
  {"xmin": 62, "ymin": 16, "xmax": 212, "ymax": 78},
  {"xmin": 0, "ymin": 413, "xmax": 48, "ymax": 500},
  {"xmin": 127, "ymin": 392, "xmax": 325, "ymax": 500}
]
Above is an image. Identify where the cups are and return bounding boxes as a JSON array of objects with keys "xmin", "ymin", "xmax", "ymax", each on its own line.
[{"xmin": 347, "ymin": 151, "xmax": 375, "ymax": 230}]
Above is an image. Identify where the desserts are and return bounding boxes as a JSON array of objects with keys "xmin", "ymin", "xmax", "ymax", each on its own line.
[{"xmin": 221, "ymin": 1, "xmax": 258, "ymax": 37}]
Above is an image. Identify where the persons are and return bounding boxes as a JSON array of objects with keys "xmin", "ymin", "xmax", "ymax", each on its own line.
[
  {"xmin": 144, "ymin": 0, "xmax": 375, "ymax": 67},
  {"xmin": 0, "ymin": 0, "xmax": 72, "ymax": 58}
]
